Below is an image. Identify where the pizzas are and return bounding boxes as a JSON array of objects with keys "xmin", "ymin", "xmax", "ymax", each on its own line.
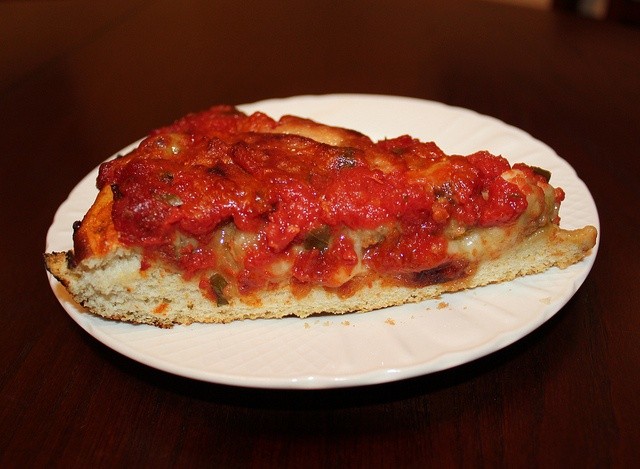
[{"xmin": 43, "ymin": 102, "xmax": 599, "ymax": 329}]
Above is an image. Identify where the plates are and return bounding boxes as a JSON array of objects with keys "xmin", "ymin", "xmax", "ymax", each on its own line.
[{"xmin": 41, "ymin": 92, "xmax": 600, "ymax": 389}]
